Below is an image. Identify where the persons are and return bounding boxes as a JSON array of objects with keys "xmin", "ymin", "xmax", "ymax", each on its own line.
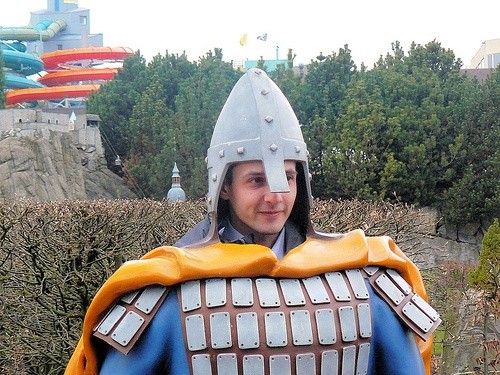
[{"xmin": 61, "ymin": 66, "xmax": 444, "ymax": 374}]
